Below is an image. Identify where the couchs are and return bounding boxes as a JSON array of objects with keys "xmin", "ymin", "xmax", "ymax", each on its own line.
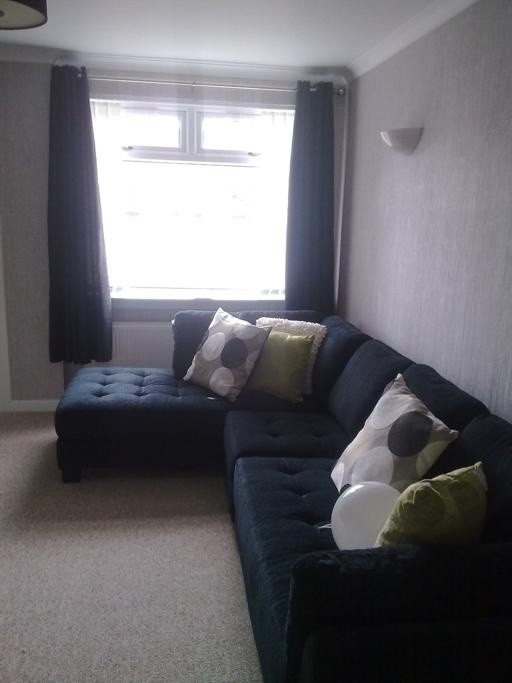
[{"xmin": 47, "ymin": 307, "xmax": 511, "ymax": 681}]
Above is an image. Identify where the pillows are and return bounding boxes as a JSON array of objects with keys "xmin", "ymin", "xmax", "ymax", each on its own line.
[
  {"xmin": 253, "ymin": 311, "xmax": 325, "ymax": 358},
  {"xmin": 373, "ymin": 456, "xmax": 489, "ymax": 552},
  {"xmin": 246, "ymin": 322, "xmax": 315, "ymax": 404},
  {"xmin": 328, "ymin": 369, "xmax": 458, "ymax": 495},
  {"xmin": 181, "ymin": 304, "xmax": 271, "ymax": 402}
]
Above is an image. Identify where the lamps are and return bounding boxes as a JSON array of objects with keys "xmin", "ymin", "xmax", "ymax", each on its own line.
[
  {"xmin": 379, "ymin": 123, "xmax": 422, "ymax": 156},
  {"xmin": 0, "ymin": 0, "xmax": 49, "ymax": 30}
]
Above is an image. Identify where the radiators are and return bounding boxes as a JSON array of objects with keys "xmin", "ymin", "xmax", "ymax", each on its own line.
[{"xmin": 82, "ymin": 318, "xmax": 177, "ymax": 370}]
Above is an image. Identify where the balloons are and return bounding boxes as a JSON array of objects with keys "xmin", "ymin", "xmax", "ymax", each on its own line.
[{"xmin": 331, "ymin": 481, "xmax": 402, "ymax": 550}]
